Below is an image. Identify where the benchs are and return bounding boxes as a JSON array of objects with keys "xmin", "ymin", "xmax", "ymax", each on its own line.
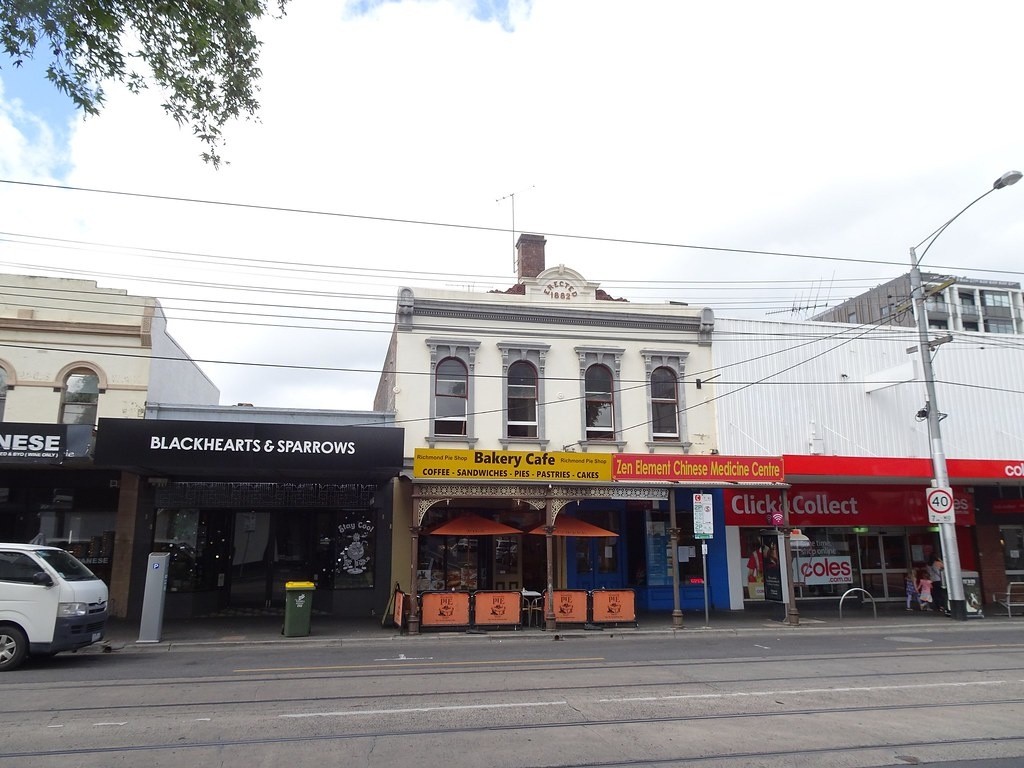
[{"xmin": 993, "ymin": 582, "xmax": 1024, "ymax": 618}]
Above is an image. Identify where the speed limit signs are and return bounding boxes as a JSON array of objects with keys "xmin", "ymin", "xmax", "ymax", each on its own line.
[{"xmin": 926, "ymin": 487, "xmax": 956, "ymax": 524}]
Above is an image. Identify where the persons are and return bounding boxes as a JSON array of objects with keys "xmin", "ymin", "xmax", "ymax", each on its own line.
[
  {"xmin": 906, "ymin": 551, "xmax": 944, "ymax": 613},
  {"xmin": 747, "ymin": 536, "xmax": 768, "ymax": 583}
]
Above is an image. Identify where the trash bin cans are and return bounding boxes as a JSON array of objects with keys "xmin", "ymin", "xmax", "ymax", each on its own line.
[{"xmin": 281, "ymin": 580, "xmax": 316, "ymax": 639}]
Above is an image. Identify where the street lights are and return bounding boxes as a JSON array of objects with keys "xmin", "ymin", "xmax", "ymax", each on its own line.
[{"xmin": 908, "ymin": 170, "xmax": 1023, "ymax": 619}]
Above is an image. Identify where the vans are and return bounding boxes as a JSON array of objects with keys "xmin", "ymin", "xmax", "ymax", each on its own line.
[{"xmin": 1, "ymin": 540, "xmax": 109, "ymax": 670}]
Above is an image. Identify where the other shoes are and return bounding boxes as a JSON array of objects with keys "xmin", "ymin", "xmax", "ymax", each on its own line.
[
  {"xmin": 927, "ymin": 608, "xmax": 933, "ymax": 611},
  {"xmin": 921, "ymin": 604, "xmax": 925, "ymax": 610},
  {"xmin": 905, "ymin": 606, "xmax": 914, "ymax": 611}
]
